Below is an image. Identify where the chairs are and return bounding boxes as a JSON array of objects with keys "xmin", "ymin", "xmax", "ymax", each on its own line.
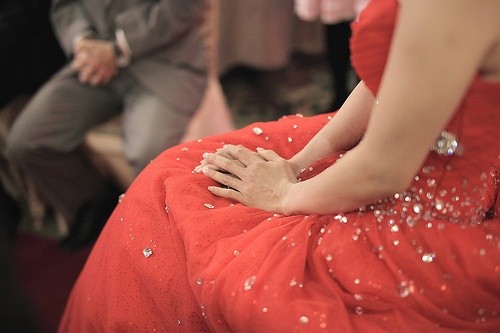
[{"xmin": 86, "ymin": 0, "xmax": 237, "ymax": 189}]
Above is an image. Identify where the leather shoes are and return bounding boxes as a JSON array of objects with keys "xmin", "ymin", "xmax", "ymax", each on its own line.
[{"xmin": 55, "ymin": 190, "xmax": 119, "ymax": 248}]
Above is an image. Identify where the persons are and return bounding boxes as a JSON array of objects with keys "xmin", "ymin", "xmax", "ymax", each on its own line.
[
  {"xmin": 56, "ymin": 0, "xmax": 500, "ymax": 333},
  {"xmin": 0, "ymin": 0, "xmax": 372, "ymax": 256}
]
